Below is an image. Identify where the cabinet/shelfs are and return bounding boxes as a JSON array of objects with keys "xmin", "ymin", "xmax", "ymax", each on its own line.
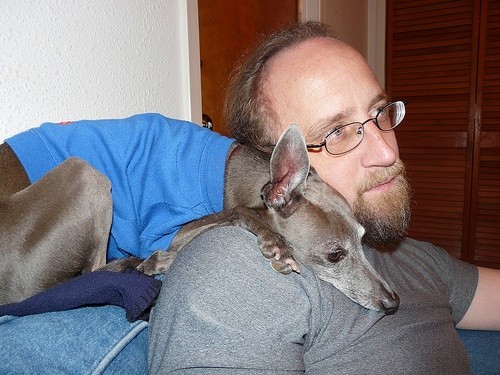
[{"xmin": 384, "ymin": 0, "xmax": 500, "ymax": 271}]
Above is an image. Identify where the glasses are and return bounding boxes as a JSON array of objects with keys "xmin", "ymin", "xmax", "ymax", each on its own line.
[{"xmin": 259, "ymin": 100, "xmax": 408, "ymax": 155}]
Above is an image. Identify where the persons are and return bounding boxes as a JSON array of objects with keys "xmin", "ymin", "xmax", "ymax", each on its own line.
[
  {"xmin": 0, "ymin": 273, "xmax": 165, "ymax": 375},
  {"xmin": 149, "ymin": 22, "xmax": 500, "ymax": 374}
]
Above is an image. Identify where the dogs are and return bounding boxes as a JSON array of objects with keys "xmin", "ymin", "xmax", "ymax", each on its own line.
[{"xmin": 0, "ymin": 113, "xmax": 401, "ymax": 314}]
